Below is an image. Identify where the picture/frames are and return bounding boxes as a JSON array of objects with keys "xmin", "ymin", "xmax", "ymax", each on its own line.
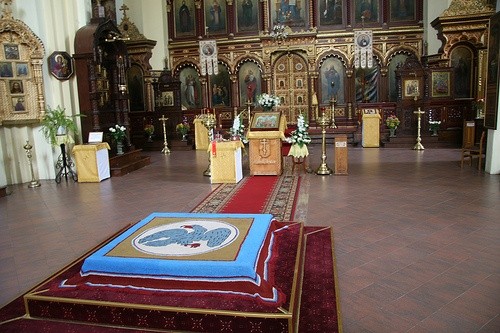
[
  {"xmin": 0, "ymin": 42, "xmax": 31, "ymax": 114},
  {"xmin": 47, "ymin": 51, "xmax": 74, "ymax": 80}
]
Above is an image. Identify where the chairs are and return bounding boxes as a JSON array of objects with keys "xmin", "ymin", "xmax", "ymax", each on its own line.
[{"xmin": 461, "ymin": 127, "xmax": 486, "ymax": 172}]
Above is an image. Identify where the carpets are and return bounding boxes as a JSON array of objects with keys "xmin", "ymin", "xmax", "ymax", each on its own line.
[{"xmin": 189, "ymin": 175, "xmax": 302, "ymax": 221}]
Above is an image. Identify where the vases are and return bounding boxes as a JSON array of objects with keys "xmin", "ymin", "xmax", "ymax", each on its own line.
[
  {"xmin": 116, "ymin": 139, "xmax": 125, "ymax": 155},
  {"xmin": 388, "ymin": 127, "xmax": 396, "ymax": 137},
  {"xmin": 181, "ymin": 134, "xmax": 188, "ymax": 141},
  {"xmin": 430, "ymin": 129, "xmax": 440, "ymax": 137}
]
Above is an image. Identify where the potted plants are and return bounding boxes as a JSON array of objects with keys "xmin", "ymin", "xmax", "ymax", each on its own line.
[{"xmin": 39, "ymin": 104, "xmax": 87, "ymax": 147}]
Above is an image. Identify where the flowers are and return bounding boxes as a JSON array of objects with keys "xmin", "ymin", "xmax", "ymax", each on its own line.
[
  {"xmin": 429, "ymin": 119, "xmax": 441, "ymax": 131},
  {"xmin": 176, "ymin": 121, "xmax": 190, "ymax": 134},
  {"xmin": 109, "ymin": 124, "xmax": 127, "ymax": 143},
  {"xmin": 256, "ymin": 93, "xmax": 281, "ymax": 112},
  {"xmin": 386, "ymin": 113, "xmax": 400, "ymax": 129},
  {"xmin": 291, "ymin": 113, "xmax": 312, "ymax": 147}
]
